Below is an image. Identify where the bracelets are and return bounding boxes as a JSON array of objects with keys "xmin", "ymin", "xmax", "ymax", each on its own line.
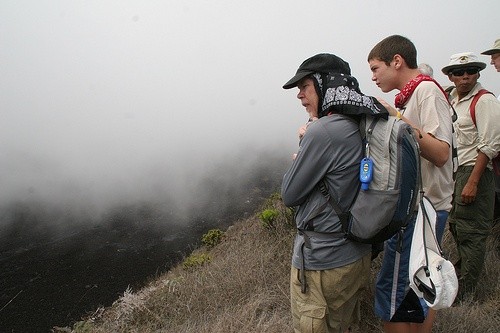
[{"xmin": 397, "ymin": 112, "xmax": 400, "ymax": 117}]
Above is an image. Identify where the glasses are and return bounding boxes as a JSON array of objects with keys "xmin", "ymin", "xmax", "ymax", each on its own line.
[{"xmin": 449, "ymin": 67, "xmax": 478, "ymax": 77}]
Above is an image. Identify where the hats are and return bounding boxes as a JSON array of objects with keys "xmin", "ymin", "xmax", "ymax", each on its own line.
[
  {"xmin": 282, "ymin": 53, "xmax": 351, "ymax": 89},
  {"xmin": 442, "ymin": 51, "xmax": 486, "ymax": 75},
  {"xmin": 482, "ymin": 39, "xmax": 500, "ymax": 56}
]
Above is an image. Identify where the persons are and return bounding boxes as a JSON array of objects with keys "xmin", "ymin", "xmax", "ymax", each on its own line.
[
  {"xmin": 282, "ymin": 53, "xmax": 372, "ymax": 333},
  {"xmin": 441, "ymin": 52, "xmax": 500, "ymax": 304},
  {"xmin": 368, "ymin": 35, "xmax": 455, "ymax": 333},
  {"xmin": 481, "ymin": 38, "xmax": 500, "ymax": 178}
]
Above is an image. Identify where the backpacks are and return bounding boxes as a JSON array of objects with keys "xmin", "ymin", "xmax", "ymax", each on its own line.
[{"xmin": 318, "ymin": 115, "xmax": 419, "ymax": 243}]
